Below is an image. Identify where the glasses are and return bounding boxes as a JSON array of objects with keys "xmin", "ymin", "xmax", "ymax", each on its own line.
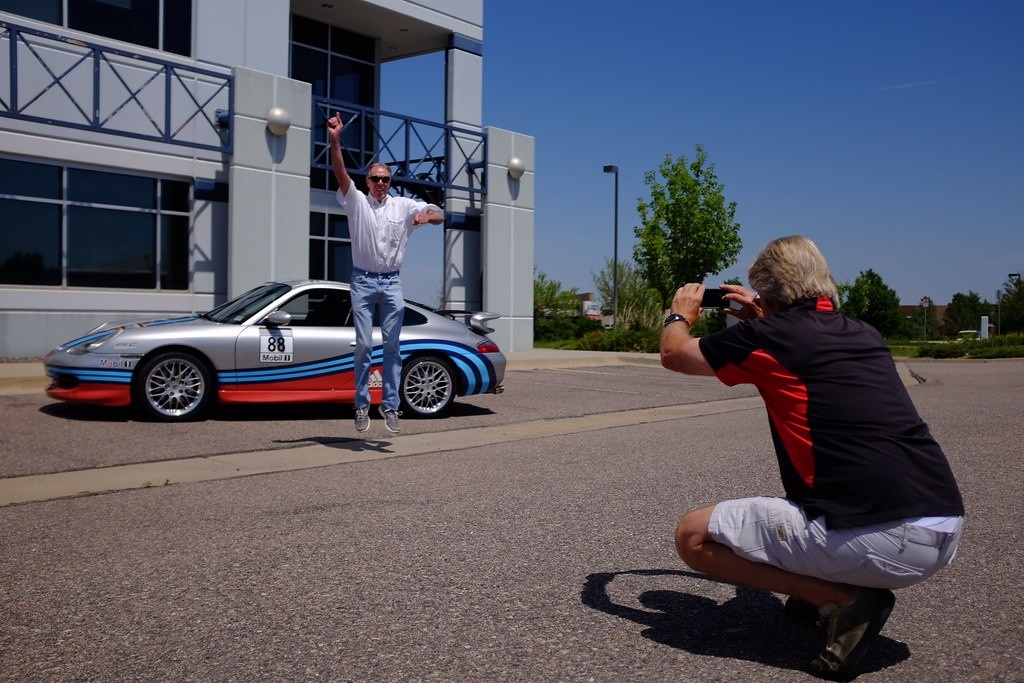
[{"xmin": 368, "ymin": 176, "xmax": 390, "ymax": 183}]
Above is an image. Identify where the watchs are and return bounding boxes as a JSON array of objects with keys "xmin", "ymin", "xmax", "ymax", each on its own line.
[{"xmin": 664, "ymin": 314, "xmax": 691, "ymax": 329}]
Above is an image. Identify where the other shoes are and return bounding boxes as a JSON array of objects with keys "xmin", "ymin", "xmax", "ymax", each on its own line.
[
  {"xmin": 807, "ymin": 587, "xmax": 896, "ymax": 682},
  {"xmin": 785, "ymin": 596, "xmax": 818, "ymax": 621}
]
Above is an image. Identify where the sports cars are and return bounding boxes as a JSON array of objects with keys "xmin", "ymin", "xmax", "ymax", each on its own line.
[{"xmin": 42, "ymin": 277, "xmax": 510, "ymax": 424}]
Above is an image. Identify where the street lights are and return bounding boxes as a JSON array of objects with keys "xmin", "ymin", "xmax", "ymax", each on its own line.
[{"xmin": 604, "ymin": 165, "xmax": 619, "ymax": 353}]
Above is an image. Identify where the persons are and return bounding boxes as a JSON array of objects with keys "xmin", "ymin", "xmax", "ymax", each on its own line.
[
  {"xmin": 327, "ymin": 112, "xmax": 446, "ymax": 433},
  {"xmin": 660, "ymin": 236, "xmax": 966, "ymax": 675}
]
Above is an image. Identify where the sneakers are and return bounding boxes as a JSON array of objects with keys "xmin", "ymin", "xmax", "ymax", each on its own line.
[
  {"xmin": 351, "ymin": 402, "xmax": 370, "ymax": 432},
  {"xmin": 378, "ymin": 404, "xmax": 403, "ymax": 433}
]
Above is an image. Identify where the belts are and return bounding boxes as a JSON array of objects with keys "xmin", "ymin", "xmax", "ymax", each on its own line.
[{"xmin": 352, "ymin": 266, "xmax": 398, "ymax": 278}]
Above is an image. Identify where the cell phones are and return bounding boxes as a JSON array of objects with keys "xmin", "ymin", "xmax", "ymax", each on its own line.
[{"xmin": 699, "ymin": 289, "xmax": 730, "ymax": 309}]
